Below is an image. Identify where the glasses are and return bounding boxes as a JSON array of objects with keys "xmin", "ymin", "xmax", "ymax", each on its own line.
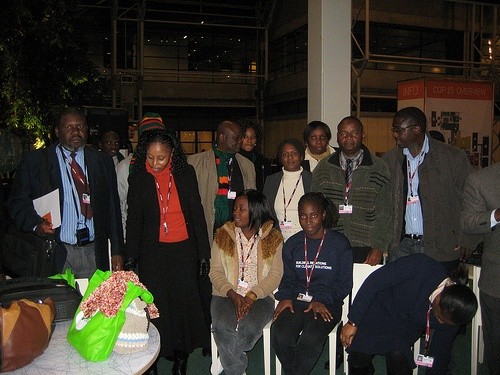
[{"xmin": 391, "ymin": 124, "xmax": 418, "ymax": 134}]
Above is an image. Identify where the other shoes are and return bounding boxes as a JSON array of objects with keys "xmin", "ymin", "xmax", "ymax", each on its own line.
[
  {"xmin": 144, "ymin": 357, "xmax": 184, "ymax": 374},
  {"xmin": 210, "ymin": 356, "xmax": 224, "ymax": 375}
]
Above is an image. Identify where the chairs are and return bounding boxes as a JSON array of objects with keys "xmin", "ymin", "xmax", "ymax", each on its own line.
[
  {"xmin": 346, "ymin": 261, "xmax": 420, "ymax": 375},
  {"xmin": 272, "ymin": 277, "xmax": 345, "ymax": 374},
  {"xmin": 455, "ymin": 263, "xmax": 486, "ymax": 374},
  {"xmin": 210, "ymin": 298, "xmax": 274, "ymax": 374}
]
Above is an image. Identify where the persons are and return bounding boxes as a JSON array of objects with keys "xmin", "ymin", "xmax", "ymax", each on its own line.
[
  {"xmin": 269, "ymin": 192, "xmax": 355, "ymax": 375},
  {"xmin": 302, "ymin": 121, "xmax": 338, "ymax": 175},
  {"xmin": 261, "ymin": 139, "xmax": 318, "ymax": 242},
  {"xmin": 237, "ymin": 121, "xmax": 271, "ymax": 177},
  {"xmin": 94, "ymin": 125, "xmax": 126, "ymax": 174},
  {"xmin": 209, "ymin": 189, "xmax": 284, "ymax": 375},
  {"xmin": 124, "ymin": 129, "xmax": 212, "ymax": 375},
  {"xmin": 114, "ymin": 112, "xmax": 166, "ymax": 244},
  {"xmin": 187, "ymin": 121, "xmax": 257, "ymax": 359},
  {"xmin": 460, "ymin": 159, "xmax": 500, "ymax": 375},
  {"xmin": 5, "ymin": 108, "xmax": 126, "ymax": 281},
  {"xmin": 311, "ymin": 114, "xmax": 395, "ymax": 265},
  {"xmin": 380, "ymin": 105, "xmax": 486, "ymax": 263},
  {"xmin": 339, "ymin": 252, "xmax": 477, "ymax": 375}
]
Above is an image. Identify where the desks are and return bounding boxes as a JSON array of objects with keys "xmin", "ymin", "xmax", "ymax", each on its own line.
[{"xmin": 3, "ymin": 272, "xmax": 160, "ymax": 375}]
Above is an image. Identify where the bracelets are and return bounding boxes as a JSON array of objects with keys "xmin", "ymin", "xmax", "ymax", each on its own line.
[{"xmin": 347, "ymin": 321, "xmax": 357, "ymax": 328}]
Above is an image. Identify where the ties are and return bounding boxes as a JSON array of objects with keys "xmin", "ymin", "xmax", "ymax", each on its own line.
[
  {"xmin": 343, "ymin": 159, "xmax": 353, "ymax": 207},
  {"xmin": 69, "ymin": 152, "xmax": 93, "ymax": 221}
]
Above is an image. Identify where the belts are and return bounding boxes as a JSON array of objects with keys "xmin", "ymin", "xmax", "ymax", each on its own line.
[{"xmin": 405, "ymin": 233, "xmax": 424, "ymax": 240}]
